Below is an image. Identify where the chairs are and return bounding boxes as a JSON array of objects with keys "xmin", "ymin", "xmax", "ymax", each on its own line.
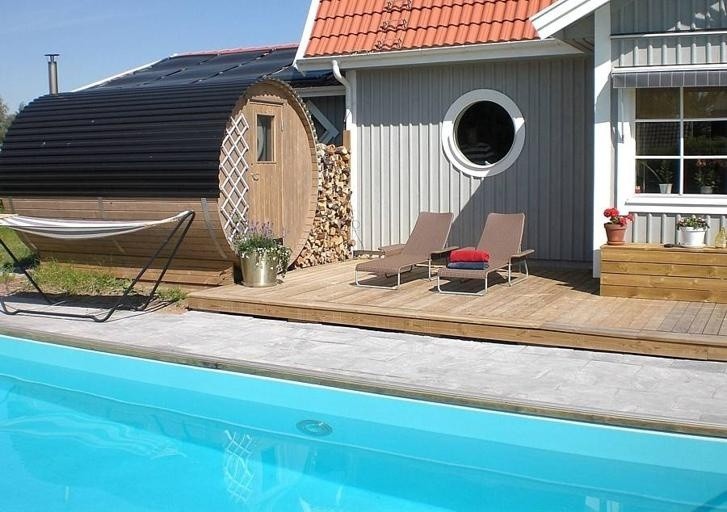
[{"xmin": 355, "ymin": 210, "xmax": 535, "ymax": 296}]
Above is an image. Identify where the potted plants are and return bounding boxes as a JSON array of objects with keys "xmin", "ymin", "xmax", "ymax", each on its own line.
[
  {"xmin": 694, "ymin": 161, "xmax": 718, "ymax": 194},
  {"xmin": 656, "ymin": 165, "xmax": 677, "ymax": 194},
  {"xmin": 675, "ymin": 216, "xmax": 710, "ymax": 248}
]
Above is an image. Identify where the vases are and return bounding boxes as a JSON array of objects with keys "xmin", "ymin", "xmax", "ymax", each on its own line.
[
  {"xmin": 240, "ymin": 250, "xmax": 281, "ymax": 288},
  {"xmin": 604, "ymin": 222, "xmax": 626, "ymax": 242}
]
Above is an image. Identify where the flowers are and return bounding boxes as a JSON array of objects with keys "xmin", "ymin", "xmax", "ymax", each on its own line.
[
  {"xmin": 603, "ymin": 205, "xmax": 634, "ymax": 225},
  {"xmin": 229, "ymin": 229, "xmax": 293, "ymax": 280}
]
